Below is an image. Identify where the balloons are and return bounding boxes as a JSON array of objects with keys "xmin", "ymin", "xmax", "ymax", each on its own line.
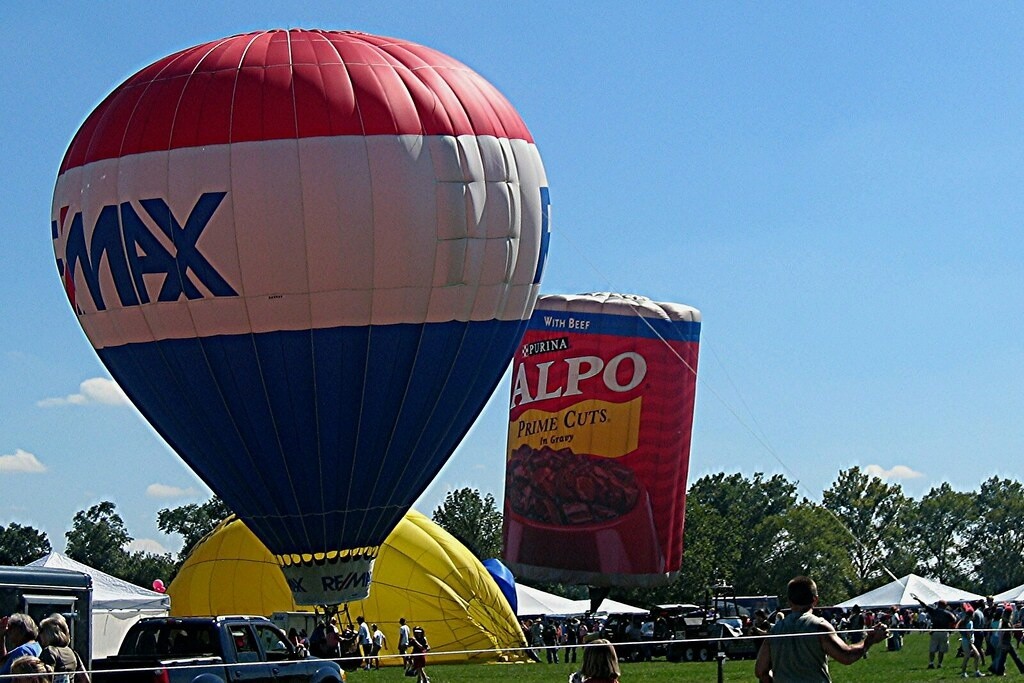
[{"xmin": 153, "ymin": 579, "xmax": 166, "ymax": 593}]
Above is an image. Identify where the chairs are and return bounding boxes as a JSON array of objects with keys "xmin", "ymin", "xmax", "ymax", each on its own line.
[
  {"xmin": 136, "ymin": 631, "xmax": 158, "ymax": 655},
  {"xmin": 169, "ymin": 631, "xmax": 203, "ymax": 655}
]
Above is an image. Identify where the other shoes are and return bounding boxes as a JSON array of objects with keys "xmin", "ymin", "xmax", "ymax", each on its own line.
[
  {"xmin": 927, "ymin": 664, "xmax": 934, "ymax": 670},
  {"xmin": 975, "ymin": 670, "xmax": 985, "ymax": 677},
  {"xmin": 937, "ymin": 664, "xmax": 942, "ymax": 668},
  {"xmin": 961, "ymin": 673, "xmax": 969, "ymax": 678}
]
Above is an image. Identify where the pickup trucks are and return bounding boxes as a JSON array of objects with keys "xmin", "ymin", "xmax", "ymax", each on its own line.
[{"xmin": 93, "ymin": 615, "xmax": 347, "ymax": 683}]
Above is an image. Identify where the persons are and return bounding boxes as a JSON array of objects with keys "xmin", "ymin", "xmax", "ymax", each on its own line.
[
  {"xmin": 288, "ymin": 615, "xmax": 388, "ymax": 671},
  {"xmin": 830, "ymin": 595, "xmax": 1024, "ymax": 677},
  {"xmin": 569, "ymin": 638, "xmax": 621, "ymax": 683},
  {"xmin": 521, "ymin": 617, "xmax": 600, "ymax": 664},
  {"xmin": 0, "ymin": 612, "xmax": 77, "ymax": 683},
  {"xmin": 409, "ymin": 626, "xmax": 430, "ymax": 683},
  {"xmin": 753, "ymin": 606, "xmax": 785, "ymax": 642},
  {"xmin": 754, "ymin": 576, "xmax": 890, "ymax": 683},
  {"xmin": 398, "ymin": 618, "xmax": 413, "ymax": 669}
]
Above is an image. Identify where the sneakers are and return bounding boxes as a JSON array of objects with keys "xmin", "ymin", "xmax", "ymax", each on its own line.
[{"xmin": 364, "ymin": 664, "xmax": 370, "ymax": 671}]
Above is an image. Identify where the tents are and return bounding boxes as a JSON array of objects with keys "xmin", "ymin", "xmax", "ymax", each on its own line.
[
  {"xmin": 514, "ymin": 582, "xmax": 651, "ymax": 616},
  {"xmin": 835, "ymin": 573, "xmax": 1024, "ymax": 606},
  {"xmin": 26, "ymin": 551, "xmax": 171, "ymax": 661}
]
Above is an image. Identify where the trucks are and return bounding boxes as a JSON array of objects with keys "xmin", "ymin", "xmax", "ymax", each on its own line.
[{"xmin": 583, "ymin": 604, "xmax": 846, "ymax": 662}]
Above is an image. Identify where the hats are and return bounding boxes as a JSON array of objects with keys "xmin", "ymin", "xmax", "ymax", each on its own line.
[
  {"xmin": 536, "ymin": 618, "xmax": 541, "ymax": 621},
  {"xmin": 371, "ymin": 624, "xmax": 377, "ymax": 626}
]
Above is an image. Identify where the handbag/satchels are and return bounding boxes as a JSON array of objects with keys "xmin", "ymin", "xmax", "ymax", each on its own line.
[{"xmin": 72, "ymin": 651, "xmax": 91, "ymax": 683}]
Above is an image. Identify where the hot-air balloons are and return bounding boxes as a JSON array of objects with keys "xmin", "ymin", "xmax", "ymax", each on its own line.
[
  {"xmin": 501, "ymin": 292, "xmax": 701, "ymax": 633},
  {"xmin": 163, "ymin": 506, "xmax": 543, "ymax": 666},
  {"xmin": 50, "ymin": 28, "xmax": 554, "ymax": 671}
]
